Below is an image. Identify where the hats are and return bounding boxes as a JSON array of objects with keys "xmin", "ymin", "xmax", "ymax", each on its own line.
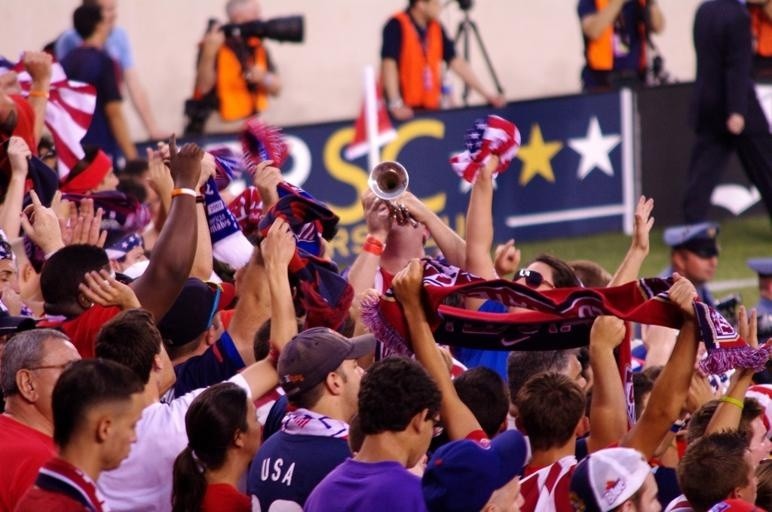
[
  {"xmin": 745, "ymin": 260, "xmax": 772, "ymax": 279},
  {"xmin": 664, "ymin": 223, "xmax": 720, "ymax": 259},
  {"xmin": 421, "ymin": 429, "xmax": 527, "ymax": 512},
  {"xmin": 570, "ymin": 447, "xmax": 651, "ymax": 512},
  {"xmin": 276, "ymin": 326, "xmax": 375, "ymax": 401},
  {"xmin": 157, "ymin": 277, "xmax": 222, "ymax": 349}
]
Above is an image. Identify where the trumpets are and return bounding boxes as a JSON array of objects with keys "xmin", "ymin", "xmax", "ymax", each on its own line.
[{"xmin": 370, "ymin": 162, "xmax": 418, "ymax": 228}]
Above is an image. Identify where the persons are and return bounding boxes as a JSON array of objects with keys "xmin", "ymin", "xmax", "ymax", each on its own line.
[
  {"xmin": 380, "ymin": 0, "xmax": 507, "ymax": 124},
  {"xmin": 681, "ymin": 2, "xmax": 771, "ymax": 224},
  {"xmin": 45, "ymin": 0, "xmax": 161, "ymax": 141},
  {"xmin": 745, "ymin": 0, "xmax": 770, "ymax": 87},
  {"xmin": 194, "ymin": 1, "xmax": 282, "ymax": 137},
  {"xmin": 577, "ymin": 1, "xmax": 666, "ymax": 93},
  {"xmin": 59, "ymin": 4, "xmax": 140, "ymax": 165},
  {"xmin": 0, "ymin": 44, "xmax": 772, "ymax": 512}
]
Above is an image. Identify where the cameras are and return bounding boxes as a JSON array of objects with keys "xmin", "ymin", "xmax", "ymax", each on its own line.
[{"xmin": 218, "ymin": 15, "xmax": 303, "ymax": 42}]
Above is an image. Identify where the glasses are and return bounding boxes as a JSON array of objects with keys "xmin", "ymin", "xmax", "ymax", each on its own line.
[
  {"xmin": 426, "ymin": 414, "xmax": 444, "ymax": 437},
  {"xmin": 513, "ymin": 269, "xmax": 554, "ymax": 289},
  {"xmin": 29, "ymin": 361, "xmax": 73, "ymax": 371}
]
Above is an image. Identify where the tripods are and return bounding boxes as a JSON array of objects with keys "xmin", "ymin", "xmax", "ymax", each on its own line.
[{"xmin": 453, "ymin": 18, "xmax": 504, "ymax": 106}]
